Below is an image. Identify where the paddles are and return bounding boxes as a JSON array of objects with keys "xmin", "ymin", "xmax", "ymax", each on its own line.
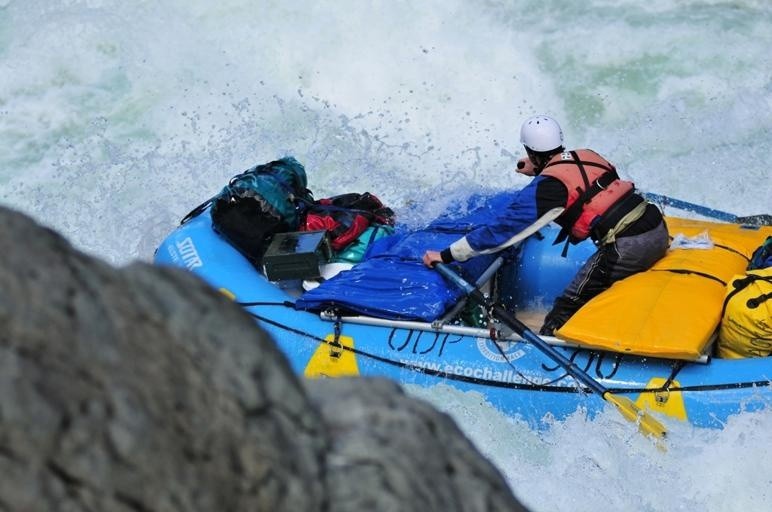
[{"xmin": 432, "ymin": 261, "xmax": 670, "ymax": 454}]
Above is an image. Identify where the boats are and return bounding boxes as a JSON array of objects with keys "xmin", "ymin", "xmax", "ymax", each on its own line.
[{"xmin": 155, "ymin": 187, "xmax": 768, "ymax": 430}]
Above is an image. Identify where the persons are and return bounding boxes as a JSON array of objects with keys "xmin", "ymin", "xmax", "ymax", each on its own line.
[{"xmin": 423, "ymin": 113, "xmax": 669, "ymax": 339}]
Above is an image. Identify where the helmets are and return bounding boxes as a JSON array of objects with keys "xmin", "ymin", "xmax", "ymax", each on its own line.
[{"xmin": 520, "ymin": 115, "xmax": 564, "ymax": 152}]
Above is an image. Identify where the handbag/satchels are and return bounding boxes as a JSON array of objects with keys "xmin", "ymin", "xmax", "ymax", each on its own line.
[{"xmin": 210, "ymin": 157, "xmax": 395, "ymax": 266}]
